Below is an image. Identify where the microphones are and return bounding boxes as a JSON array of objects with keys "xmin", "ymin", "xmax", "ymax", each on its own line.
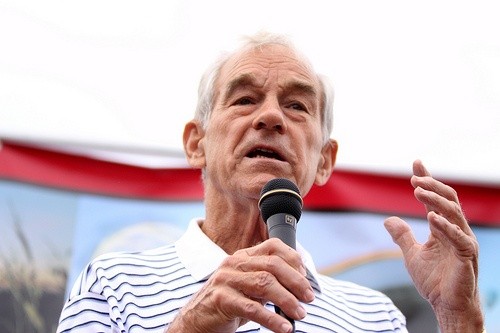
[{"xmin": 258, "ymin": 178, "xmax": 303, "ymax": 333}]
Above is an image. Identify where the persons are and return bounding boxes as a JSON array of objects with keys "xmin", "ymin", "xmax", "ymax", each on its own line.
[{"xmin": 55, "ymin": 32, "xmax": 484, "ymax": 333}]
